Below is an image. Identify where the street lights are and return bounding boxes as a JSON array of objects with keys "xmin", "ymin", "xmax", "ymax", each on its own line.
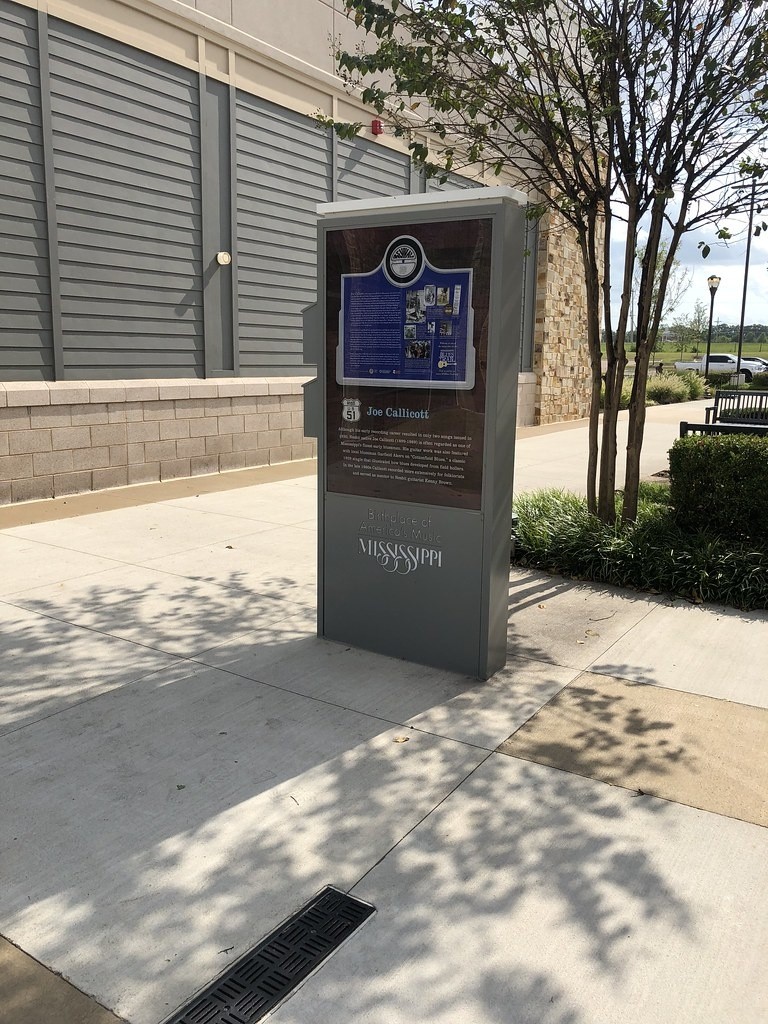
[{"xmin": 705, "ymin": 274, "xmax": 721, "ymax": 385}]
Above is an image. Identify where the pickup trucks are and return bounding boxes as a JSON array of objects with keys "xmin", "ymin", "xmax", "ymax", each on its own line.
[{"xmin": 674, "ymin": 352, "xmax": 764, "ymax": 383}]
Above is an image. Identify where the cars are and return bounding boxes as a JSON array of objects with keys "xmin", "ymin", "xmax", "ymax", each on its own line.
[{"xmin": 741, "ymin": 357, "xmax": 768, "ymax": 370}]
